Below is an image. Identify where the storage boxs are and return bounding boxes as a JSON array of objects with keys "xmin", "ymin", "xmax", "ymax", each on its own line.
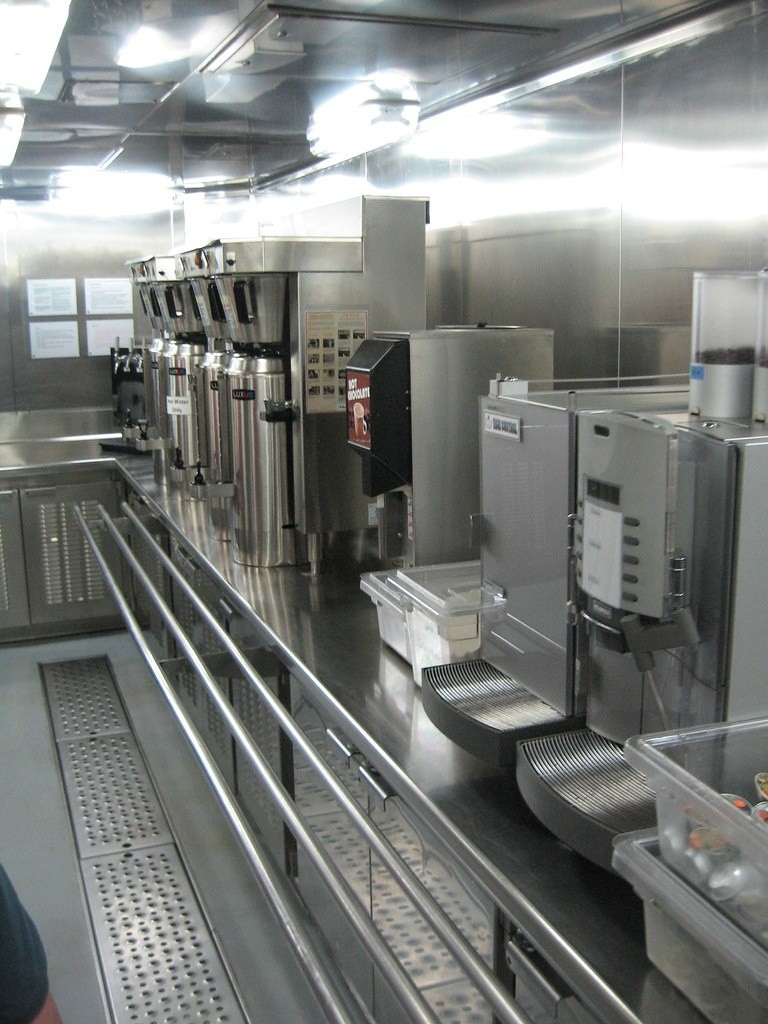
[
  {"xmin": 358, "ymin": 559, "xmax": 483, "ymax": 690},
  {"xmin": 611, "ymin": 715, "xmax": 768, "ymax": 1023}
]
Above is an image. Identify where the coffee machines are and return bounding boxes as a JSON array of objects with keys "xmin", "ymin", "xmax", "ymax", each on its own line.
[{"xmin": 342, "ymin": 324, "xmax": 555, "ymax": 608}]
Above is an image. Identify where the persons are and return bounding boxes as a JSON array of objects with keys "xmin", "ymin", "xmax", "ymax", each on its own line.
[{"xmin": 0, "ymin": 863, "xmax": 63, "ymax": 1024}]
